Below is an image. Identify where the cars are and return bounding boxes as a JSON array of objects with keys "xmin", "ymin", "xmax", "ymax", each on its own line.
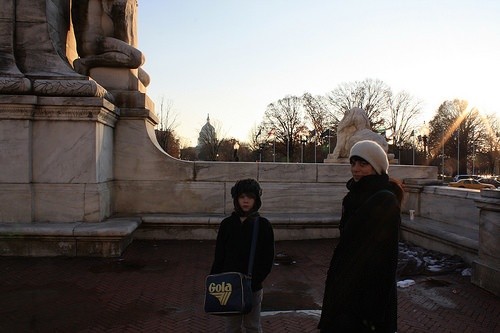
[{"xmin": 438, "ymin": 173, "xmax": 500, "ymax": 190}]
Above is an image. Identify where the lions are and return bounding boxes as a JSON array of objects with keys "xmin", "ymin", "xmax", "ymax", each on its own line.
[{"xmin": 327, "ymin": 107, "xmax": 388, "ymax": 157}]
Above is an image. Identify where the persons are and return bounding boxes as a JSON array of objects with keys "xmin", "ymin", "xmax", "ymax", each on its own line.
[
  {"xmin": 210, "ymin": 178, "xmax": 274, "ymax": 333},
  {"xmin": 315, "ymin": 140, "xmax": 404, "ymax": 333}
]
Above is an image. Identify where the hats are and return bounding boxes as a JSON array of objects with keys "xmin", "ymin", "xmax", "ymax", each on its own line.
[
  {"xmin": 231, "ymin": 179, "xmax": 262, "ymax": 216},
  {"xmin": 349, "ymin": 140, "xmax": 388, "ymax": 177}
]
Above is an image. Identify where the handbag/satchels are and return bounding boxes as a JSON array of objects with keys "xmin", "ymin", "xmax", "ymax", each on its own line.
[{"xmin": 204, "ymin": 272, "xmax": 252, "ymax": 314}]
